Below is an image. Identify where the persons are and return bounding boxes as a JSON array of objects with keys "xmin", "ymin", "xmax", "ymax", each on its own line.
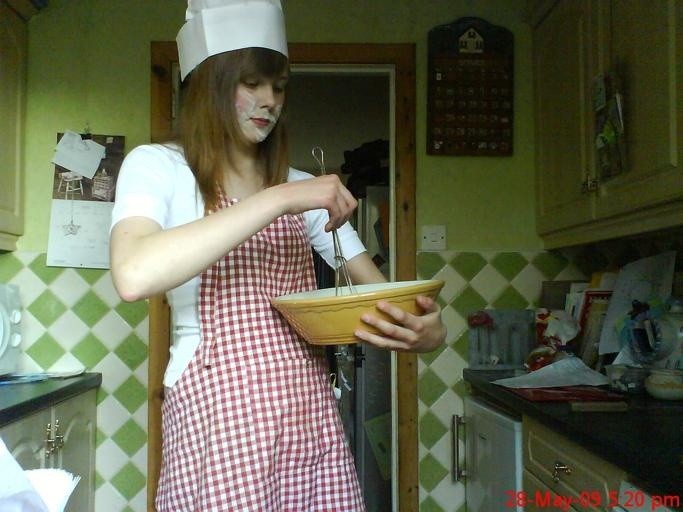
[{"xmin": 108, "ymin": 44, "xmax": 448, "ymax": 512}]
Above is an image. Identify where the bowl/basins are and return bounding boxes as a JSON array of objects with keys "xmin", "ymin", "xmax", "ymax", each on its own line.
[{"xmin": 268, "ymin": 279, "xmax": 447, "ymax": 347}]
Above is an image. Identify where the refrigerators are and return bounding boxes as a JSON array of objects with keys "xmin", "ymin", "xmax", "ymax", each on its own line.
[{"xmin": 339, "ymin": 182, "xmax": 392, "ymax": 512}]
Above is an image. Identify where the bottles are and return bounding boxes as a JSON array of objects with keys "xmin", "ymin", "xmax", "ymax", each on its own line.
[{"xmin": 646, "ymin": 369, "xmax": 683, "ymax": 401}]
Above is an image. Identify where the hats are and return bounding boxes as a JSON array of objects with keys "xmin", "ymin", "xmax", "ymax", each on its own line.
[{"xmin": 175, "ymin": 1, "xmax": 291, "ymax": 82}]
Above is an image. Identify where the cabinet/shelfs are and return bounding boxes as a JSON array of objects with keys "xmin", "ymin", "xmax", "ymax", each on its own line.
[
  {"xmin": 528, "ymin": 0, "xmax": 683, "ymax": 247},
  {"xmin": 1, "ymin": 387, "xmax": 100, "ymax": 512},
  {"xmin": 462, "ymin": 385, "xmax": 682, "ymax": 509},
  {"xmin": 0, "ymin": 5, "xmax": 27, "ymax": 254}
]
